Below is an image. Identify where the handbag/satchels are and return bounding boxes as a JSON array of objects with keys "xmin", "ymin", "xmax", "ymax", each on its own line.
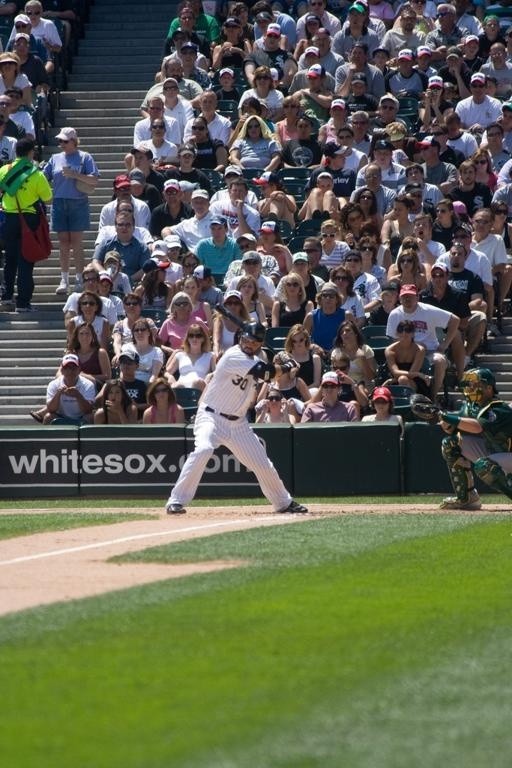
[
  {"xmin": 74, "ymin": 150, "xmax": 97, "ymax": 194},
  {"xmin": 20, "ymin": 193, "xmax": 54, "ymax": 261}
]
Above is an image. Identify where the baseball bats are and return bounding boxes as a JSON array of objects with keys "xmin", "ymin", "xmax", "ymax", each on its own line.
[{"xmin": 214, "ymin": 303, "xmax": 296, "ymax": 379}]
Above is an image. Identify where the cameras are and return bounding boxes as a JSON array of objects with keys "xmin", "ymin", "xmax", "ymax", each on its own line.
[{"xmin": 425, "ymin": 88, "xmax": 438, "ymax": 98}]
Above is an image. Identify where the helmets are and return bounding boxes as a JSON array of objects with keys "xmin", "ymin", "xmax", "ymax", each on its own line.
[
  {"xmin": 239, "ymin": 320, "xmax": 266, "ymax": 343},
  {"xmin": 459, "ymin": 366, "xmax": 499, "ymax": 403}
]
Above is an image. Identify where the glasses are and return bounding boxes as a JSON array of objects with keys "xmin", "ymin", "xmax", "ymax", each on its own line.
[
  {"xmin": 474, "ymin": 132, "xmax": 502, "ymax": 165},
  {"xmin": 188, "ymin": 333, "xmax": 201, "ymax": 339},
  {"xmin": 380, "ymin": 104, "xmax": 394, "ymax": 111},
  {"xmin": 398, "ymin": 326, "xmax": 410, "ymax": 333},
  {"xmin": 117, "ymin": 205, "xmax": 135, "ymax": 228},
  {"xmin": 322, "ymin": 233, "xmax": 337, "ymax": 238},
  {"xmin": 335, "ymin": 276, "xmax": 348, "ymax": 281},
  {"xmin": 432, "ymin": 274, "xmax": 444, "ymax": 279},
  {"xmin": 269, "ymin": 396, "xmax": 281, "ymax": 401},
  {"xmin": 338, "ymin": 134, "xmax": 351, "ymax": 140},
  {"xmin": 286, "ymin": 282, "xmax": 298, "ymax": 287},
  {"xmin": 435, "ymin": 207, "xmax": 508, "ymax": 216},
  {"xmin": 0, "ymin": 101, "xmax": 8, "ymax": 106},
  {"xmin": 304, "ymin": 248, "xmax": 319, "ymax": 254},
  {"xmin": 14, "ymin": 9, "xmax": 40, "ymax": 30},
  {"xmin": 190, "ymin": 123, "xmax": 204, "ymax": 131},
  {"xmin": 322, "ymin": 293, "xmax": 336, "ymax": 299},
  {"xmin": 173, "ymin": 300, "xmax": 239, "ymax": 308},
  {"xmin": 292, "ymin": 337, "xmax": 304, "ymax": 343},
  {"xmin": 283, "ymin": 104, "xmax": 296, "ymax": 109},
  {"xmin": 80, "ymin": 278, "xmax": 156, "ymax": 333},
  {"xmin": 149, "ymin": 105, "xmax": 165, "ymax": 133},
  {"xmin": 346, "ymin": 246, "xmax": 374, "ymax": 263},
  {"xmin": 153, "ymin": 389, "xmax": 168, "ymax": 393},
  {"xmin": 453, "ymin": 242, "xmax": 465, "ymax": 247},
  {"xmin": 361, "ymin": 195, "xmax": 371, "ymax": 199},
  {"xmin": 400, "ymin": 245, "xmax": 417, "ymax": 264},
  {"xmin": 164, "ymin": 85, "xmax": 174, "ymax": 90},
  {"xmin": 323, "ymin": 383, "xmax": 336, "ymax": 389}
]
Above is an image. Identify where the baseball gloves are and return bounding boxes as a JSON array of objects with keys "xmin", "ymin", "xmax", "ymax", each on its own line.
[{"xmin": 409, "ymin": 394, "xmax": 440, "ymax": 424}]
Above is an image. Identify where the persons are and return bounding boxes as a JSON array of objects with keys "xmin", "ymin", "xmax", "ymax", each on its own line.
[
  {"xmin": 29, "ymin": 162, "xmax": 512, "ymax": 423},
  {"xmin": 124, "ymin": 0, "xmax": 512, "ymax": 199},
  {"xmin": 1, "ymin": 0, "xmax": 75, "ymax": 165},
  {"xmin": 0, "ymin": 137, "xmax": 54, "ymax": 312},
  {"xmin": 409, "ymin": 366, "xmax": 511, "ymax": 510},
  {"xmin": 41, "ymin": 127, "xmax": 100, "ymax": 294},
  {"xmin": 165, "ymin": 323, "xmax": 305, "ymax": 514}
]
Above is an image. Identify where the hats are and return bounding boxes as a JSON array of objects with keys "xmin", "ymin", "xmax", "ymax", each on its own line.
[
  {"xmin": 347, "ymin": 0, "xmax": 370, "ymax": 15},
  {"xmin": 321, "ymin": 371, "xmax": 339, "ymax": 385},
  {"xmin": 430, "ymin": 262, "xmax": 450, "ymax": 273},
  {"xmin": 143, "ymin": 233, "xmax": 184, "ymax": 273},
  {"xmin": 374, "ymin": 122, "xmax": 439, "ymax": 149},
  {"xmin": 59, "ymin": 347, "xmax": 139, "ymax": 367},
  {"xmin": 223, "ymin": 289, "xmax": 243, "ymax": 302},
  {"xmin": 250, "ymin": 11, "xmax": 283, "ymax": 82},
  {"xmin": 112, "ymin": 144, "xmax": 154, "ymax": 193},
  {"xmin": 163, "ymin": 177, "xmax": 209, "ymax": 201},
  {"xmin": 0, "ymin": 14, "xmax": 32, "ymax": 98},
  {"xmin": 372, "ymin": 386, "xmax": 392, "ymax": 403},
  {"xmin": 209, "ymin": 215, "xmax": 280, "ymax": 264},
  {"xmin": 55, "ymin": 124, "xmax": 78, "ymax": 145},
  {"xmin": 191, "ymin": 263, "xmax": 211, "ymax": 280},
  {"xmin": 451, "ymin": 200, "xmax": 467, "ymax": 214},
  {"xmin": 322, "ymin": 138, "xmax": 347, "ymax": 156},
  {"xmin": 99, "ymin": 272, "xmax": 112, "ymax": 283},
  {"xmin": 103, "ymin": 249, "xmax": 121, "ymax": 264},
  {"xmin": 452, "ymin": 221, "xmax": 474, "ymax": 234},
  {"xmin": 291, "ymin": 251, "xmax": 311, "ymax": 265},
  {"xmin": 321, "ymin": 281, "xmax": 340, "ymax": 293},
  {"xmin": 176, "ymin": 142, "xmax": 278, "ymax": 185},
  {"xmin": 301, "ymin": 12, "xmax": 331, "ymax": 81},
  {"xmin": 404, "ymin": 180, "xmax": 424, "ymax": 194},
  {"xmin": 162, "ymin": 14, "xmax": 242, "ymax": 83},
  {"xmin": 331, "ymin": 73, "xmax": 399, "ymax": 108},
  {"xmin": 398, "ymin": 33, "xmax": 487, "ymax": 87},
  {"xmin": 382, "ymin": 281, "xmax": 417, "ymax": 297}
]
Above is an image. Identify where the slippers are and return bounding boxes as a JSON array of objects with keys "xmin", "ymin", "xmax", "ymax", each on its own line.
[{"xmin": 29, "ymin": 409, "xmax": 44, "ymax": 423}]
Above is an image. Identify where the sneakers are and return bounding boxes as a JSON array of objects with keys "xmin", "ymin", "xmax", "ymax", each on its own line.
[
  {"xmin": 453, "ymin": 353, "xmax": 472, "ymax": 371},
  {"xmin": 486, "ymin": 321, "xmax": 506, "ymax": 341},
  {"xmin": 268, "ymin": 210, "xmax": 278, "ymax": 220},
  {"xmin": 72, "ymin": 280, "xmax": 85, "ymax": 292},
  {"xmin": 0, "ymin": 292, "xmax": 14, "ymax": 304},
  {"xmin": 311, "ymin": 208, "xmax": 321, "ymax": 218},
  {"xmin": 278, "ymin": 501, "xmax": 309, "ymax": 513},
  {"xmin": 12, "ymin": 302, "xmax": 39, "ymax": 315},
  {"xmin": 442, "ymin": 487, "xmax": 482, "ymax": 511},
  {"xmin": 56, "ymin": 279, "xmax": 71, "ymax": 294},
  {"xmin": 166, "ymin": 502, "xmax": 188, "ymax": 514},
  {"xmin": 323, "ymin": 209, "xmax": 329, "ymax": 220}
]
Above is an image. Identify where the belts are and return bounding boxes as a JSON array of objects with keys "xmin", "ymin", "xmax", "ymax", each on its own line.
[{"xmin": 205, "ymin": 406, "xmax": 239, "ymax": 421}]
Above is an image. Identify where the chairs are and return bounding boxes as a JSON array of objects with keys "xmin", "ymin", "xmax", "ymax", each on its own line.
[
  {"xmin": 0, "ymin": 1, "xmax": 77, "ymax": 219},
  {"xmin": 54, "ymin": 1, "xmax": 512, "ymax": 423}
]
